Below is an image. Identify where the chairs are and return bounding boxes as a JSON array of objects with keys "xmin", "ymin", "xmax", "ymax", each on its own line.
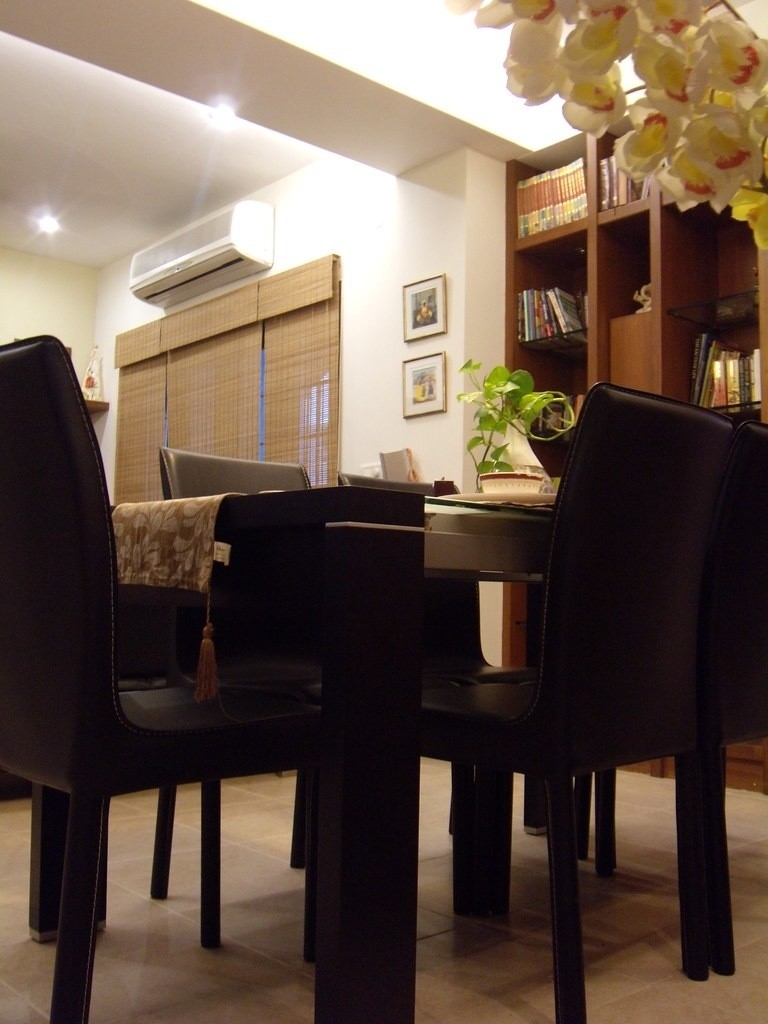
[
  {"xmin": 0, "ymin": 335, "xmax": 317, "ymax": 1024},
  {"xmin": 336, "ymin": 474, "xmax": 455, "ymax": 837},
  {"xmin": 151, "ymin": 446, "xmax": 317, "ymax": 896},
  {"xmin": 417, "ymin": 381, "xmax": 767, "ymax": 1024}
]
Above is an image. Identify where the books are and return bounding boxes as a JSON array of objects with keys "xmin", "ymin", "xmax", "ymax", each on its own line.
[
  {"xmin": 517, "ymin": 287, "xmax": 587, "ymax": 346},
  {"xmin": 599, "ymin": 156, "xmax": 654, "ymax": 210},
  {"xmin": 691, "ymin": 334, "xmax": 763, "ymax": 413},
  {"xmin": 518, "ymin": 158, "xmax": 587, "ymax": 238}
]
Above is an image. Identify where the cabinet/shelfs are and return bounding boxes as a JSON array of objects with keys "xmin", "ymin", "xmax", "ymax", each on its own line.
[{"xmin": 500, "ymin": 128, "xmax": 767, "ymax": 794}]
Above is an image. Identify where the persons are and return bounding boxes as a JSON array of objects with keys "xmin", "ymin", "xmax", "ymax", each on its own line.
[
  {"xmin": 416, "ymin": 300, "xmax": 433, "ymax": 321},
  {"xmin": 414, "ymin": 375, "xmax": 436, "ymax": 403}
]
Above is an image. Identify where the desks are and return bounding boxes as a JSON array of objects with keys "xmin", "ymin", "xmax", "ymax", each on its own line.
[{"xmin": 111, "ymin": 485, "xmax": 555, "ymax": 1024}]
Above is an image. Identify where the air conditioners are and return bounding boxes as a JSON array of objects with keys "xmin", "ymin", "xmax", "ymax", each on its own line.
[{"xmin": 130, "ymin": 201, "xmax": 275, "ymax": 310}]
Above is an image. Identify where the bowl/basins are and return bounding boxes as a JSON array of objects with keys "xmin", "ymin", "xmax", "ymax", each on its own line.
[{"xmin": 479, "ymin": 472, "xmax": 544, "ymax": 493}]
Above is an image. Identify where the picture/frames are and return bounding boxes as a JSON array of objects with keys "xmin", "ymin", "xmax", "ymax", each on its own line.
[
  {"xmin": 403, "ymin": 272, "xmax": 447, "ymax": 343},
  {"xmin": 401, "ymin": 351, "xmax": 447, "ymax": 417}
]
